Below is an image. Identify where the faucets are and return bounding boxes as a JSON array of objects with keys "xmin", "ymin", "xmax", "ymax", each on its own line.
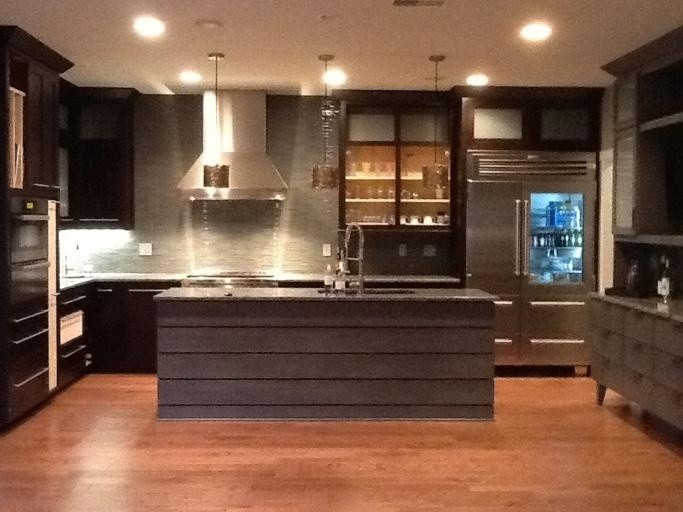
[{"xmin": 344, "ymin": 219, "xmax": 365, "ymax": 295}]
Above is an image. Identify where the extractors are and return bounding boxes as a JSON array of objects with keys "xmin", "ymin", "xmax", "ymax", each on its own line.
[{"xmin": 175, "ymin": 90, "xmax": 289, "ymax": 202}]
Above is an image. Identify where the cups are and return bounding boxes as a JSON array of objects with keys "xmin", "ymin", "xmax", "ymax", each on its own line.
[
  {"xmin": 361, "ymin": 161, "xmax": 395, "ymax": 176},
  {"xmin": 400, "ymin": 215, "xmax": 435, "ymax": 225}
]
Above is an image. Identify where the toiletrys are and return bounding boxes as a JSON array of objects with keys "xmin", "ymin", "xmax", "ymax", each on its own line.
[
  {"xmin": 324, "ymin": 264, "xmax": 334, "ymax": 297},
  {"xmin": 336, "ymin": 276, "xmax": 346, "ymax": 296}
]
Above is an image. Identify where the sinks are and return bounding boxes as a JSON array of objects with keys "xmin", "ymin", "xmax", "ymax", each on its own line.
[{"xmin": 317, "ymin": 284, "xmax": 416, "ymax": 295}]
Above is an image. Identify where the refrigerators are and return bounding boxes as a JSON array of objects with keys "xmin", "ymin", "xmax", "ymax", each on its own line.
[{"xmin": 462, "ymin": 174, "xmax": 601, "ymax": 377}]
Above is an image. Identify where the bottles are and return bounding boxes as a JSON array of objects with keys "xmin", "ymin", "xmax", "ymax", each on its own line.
[{"xmin": 656, "ymin": 253, "xmax": 670, "ymax": 299}]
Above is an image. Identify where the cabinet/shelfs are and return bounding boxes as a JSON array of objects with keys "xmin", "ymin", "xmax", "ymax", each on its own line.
[
  {"xmin": 1, "ymin": 47, "xmax": 60, "ymax": 200},
  {"xmin": 59, "ymin": 89, "xmax": 134, "ymax": 230},
  {"xmin": 336, "ymin": 98, "xmax": 467, "ymax": 289},
  {"xmin": 585, "ymin": 26, "xmax": 682, "ymax": 437},
  {"xmin": 58, "ymin": 282, "xmax": 182, "ymax": 392}
]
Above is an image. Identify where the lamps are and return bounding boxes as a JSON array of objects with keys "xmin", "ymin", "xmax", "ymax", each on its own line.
[
  {"xmin": 204, "ymin": 50, "xmax": 230, "ymax": 189},
  {"xmin": 312, "ymin": 53, "xmax": 341, "ymax": 190},
  {"xmin": 424, "ymin": 53, "xmax": 448, "ymax": 191}
]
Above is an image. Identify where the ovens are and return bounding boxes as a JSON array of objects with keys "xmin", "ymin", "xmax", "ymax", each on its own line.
[{"xmin": 9, "ymin": 195, "xmax": 52, "ymax": 266}]
